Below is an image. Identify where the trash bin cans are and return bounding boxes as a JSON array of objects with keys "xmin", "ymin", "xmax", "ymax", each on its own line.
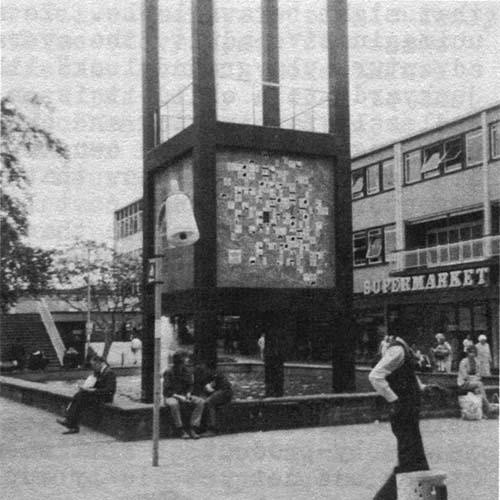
[{"xmin": 395, "ymin": 470, "xmax": 448, "ymax": 500}]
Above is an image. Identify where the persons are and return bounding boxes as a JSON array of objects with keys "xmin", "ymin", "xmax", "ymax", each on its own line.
[
  {"xmin": 26, "ymin": 344, "xmax": 50, "ymax": 370},
  {"xmin": 60, "ymin": 344, "xmax": 82, "ymax": 369},
  {"xmin": 473, "ymin": 333, "xmax": 494, "ymax": 377},
  {"xmin": 457, "ymin": 345, "xmax": 498, "ymax": 418},
  {"xmin": 370, "ymin": 317, "xmax": 430, "ymax": 499},
  {"xmin": 192, "ymin": 357, "xmax": 234, "ymax": 437},
  {"xmin": 56, "ymin": 356, "xmax": 117, "ymax": 436},
  {"xmin": 461, "ymin": 331, "xmax": 473, "ymax": 358},
  {"xmin": 161, "ymin": 349, "xmax": 205, "ymax": 440},
  {"xmin": 131, "ymin": 334, "xmax": 142, "ymax": 366},
  {"xmin": 9, "ymin": 336, "xmax": 27, "ymax": 373},
  {"xmin": 407, "ymin": 331, "xmax": 454, "ymax": 372}
]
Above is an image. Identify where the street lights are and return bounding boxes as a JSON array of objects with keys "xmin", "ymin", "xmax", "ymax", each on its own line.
[{"xmin": 152, "ymin": 193, "xmax": 200, "ymax": 466}]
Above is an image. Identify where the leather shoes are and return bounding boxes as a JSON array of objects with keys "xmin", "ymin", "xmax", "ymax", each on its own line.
[
  {"xmin": 181, "ymin": 429, "xmax": 215, "ymax": 439},
  {"xmin": 56, "ymin": 418, "xmax": 79, "ymax": 434}
]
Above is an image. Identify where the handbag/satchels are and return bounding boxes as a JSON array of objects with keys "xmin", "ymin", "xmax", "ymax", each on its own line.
[{"xmin": 458, "ymin": 392, "xmax": 483, "ymax": 420}]
